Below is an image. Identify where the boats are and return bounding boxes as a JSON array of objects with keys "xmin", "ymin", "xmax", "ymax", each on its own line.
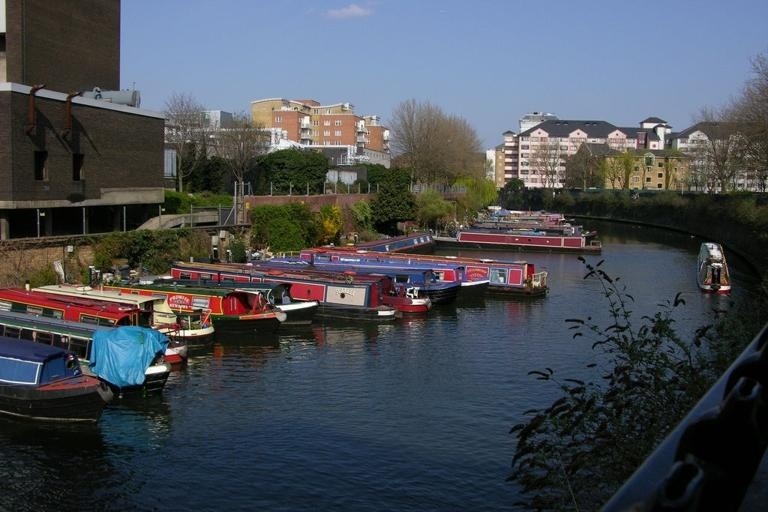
[
  {"xmin": 172, "ymin": 235, "xmax": 552, "ymax": 322},
  {"xmin": 696, "ymin": 241, "xmax": 731, "ymax": 297},
  {"xmin": 0, "ymin": 337, "xmax": 113, "ymax": 425},
  {"xmin": 148, "ymin": 275, "xmax": 320, "ymax": 327},
  {"xmin": 94, "ymin": 283, "xmax": 287, "ymax": 336},
  {"xmin": 33, "ymin": 283, "xmax": 215, "ymax": 343},
  {"xmin": 0, "ymin": 287, "xmax": 189, "ymax": 367},
  {"xmin": 0, "ymin": 311, "xmax": 172, "ymax": 399},
  {"xmin": 456, "ymin": 206, "xmax": 602, "ymax": 255}
]
